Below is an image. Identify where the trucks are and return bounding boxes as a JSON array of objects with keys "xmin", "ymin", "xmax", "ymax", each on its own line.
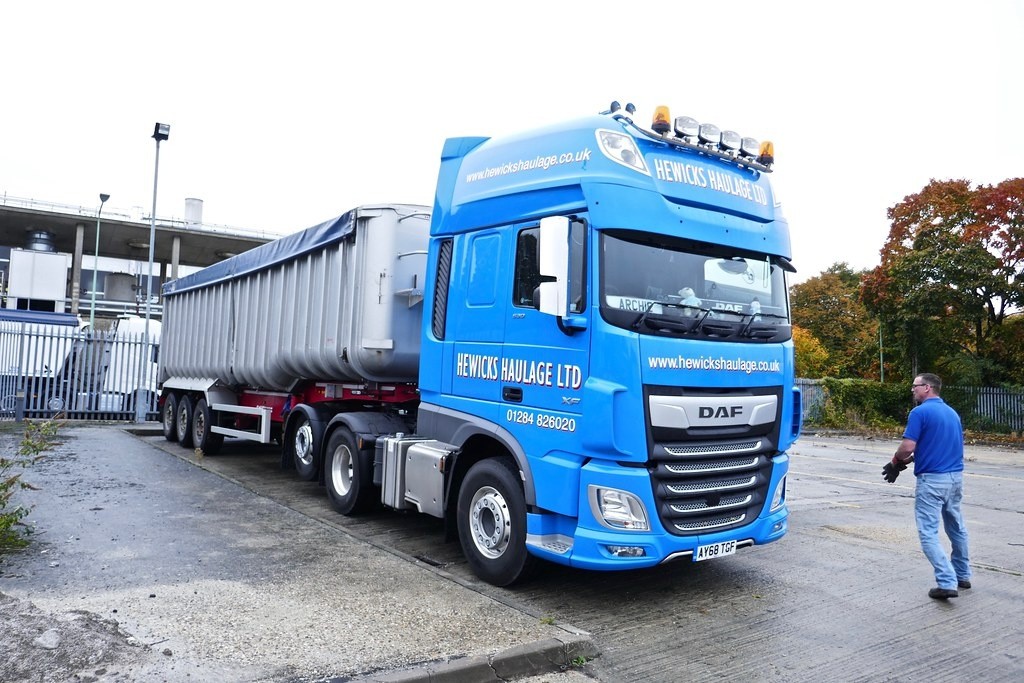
[{"xmin": 0, "ymin": 308, "xmax": 163, "ymax": 421}]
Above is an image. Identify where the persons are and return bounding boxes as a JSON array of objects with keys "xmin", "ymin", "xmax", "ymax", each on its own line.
[{"xmin": 882, "ymin": 372, "xmax": 970, "ymax": 599}]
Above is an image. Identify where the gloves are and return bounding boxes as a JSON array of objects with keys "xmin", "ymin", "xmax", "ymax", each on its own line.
[{"xmin": 881, "ymin": 455, "xmax": 907, "ymax": 483}]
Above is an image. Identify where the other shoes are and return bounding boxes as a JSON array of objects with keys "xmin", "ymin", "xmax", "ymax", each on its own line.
[
  {"xmin": 928, "ymin": 587, "xmax": 958, "ymax": 598},
  {"xmin": 958, "ymin": 581, "xmax": 971, "ymax": 588}
]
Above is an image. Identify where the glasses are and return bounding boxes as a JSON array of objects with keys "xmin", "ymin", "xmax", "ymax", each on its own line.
[{"xmin": 910, "ymin": 384, "xmax": 926, "ymax": 388}]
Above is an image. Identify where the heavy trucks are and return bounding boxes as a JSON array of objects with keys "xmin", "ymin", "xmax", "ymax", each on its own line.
[{"xmin": 154, "ymin": 101, "xmax": 802, "ymax": 587}]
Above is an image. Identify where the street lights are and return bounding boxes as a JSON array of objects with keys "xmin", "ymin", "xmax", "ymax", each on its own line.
[
  {"xmin": 135, "ymin": 121, "xmax": 169, "ymax": 425},
  {"xmin": 89, "ymin": 193, "xmax": 110, "ymax": 333}
]
